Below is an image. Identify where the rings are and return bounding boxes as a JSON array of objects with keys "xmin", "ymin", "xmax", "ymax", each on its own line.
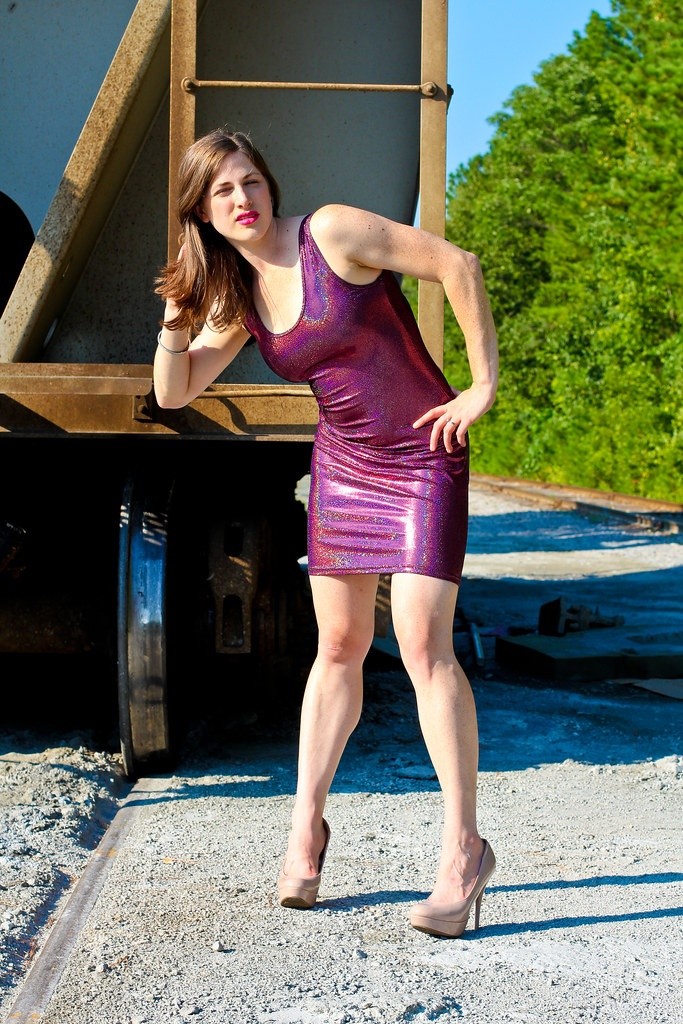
[{"xmin": 448, "ymin": 419, "xmax": 457, "ymax": 428}]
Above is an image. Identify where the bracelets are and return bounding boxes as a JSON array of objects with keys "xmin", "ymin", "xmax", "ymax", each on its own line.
[{"xmin": 156, "ymin": 329, "xmax": 192, "ymax": 356}]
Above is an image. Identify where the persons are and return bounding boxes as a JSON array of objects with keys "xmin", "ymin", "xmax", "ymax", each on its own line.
[{"xmin": 153, "ymin": 121, "xmax": 502, "ymax": 940}]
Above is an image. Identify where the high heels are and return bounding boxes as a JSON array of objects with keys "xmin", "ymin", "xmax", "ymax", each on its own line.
[
  {"xmin": 408, "ymin": 839, "xmax": 495, "ymax": 936},
  {"xmin": 275, "ymin": 812, "xmax": 330, "ymax": 909}
]
